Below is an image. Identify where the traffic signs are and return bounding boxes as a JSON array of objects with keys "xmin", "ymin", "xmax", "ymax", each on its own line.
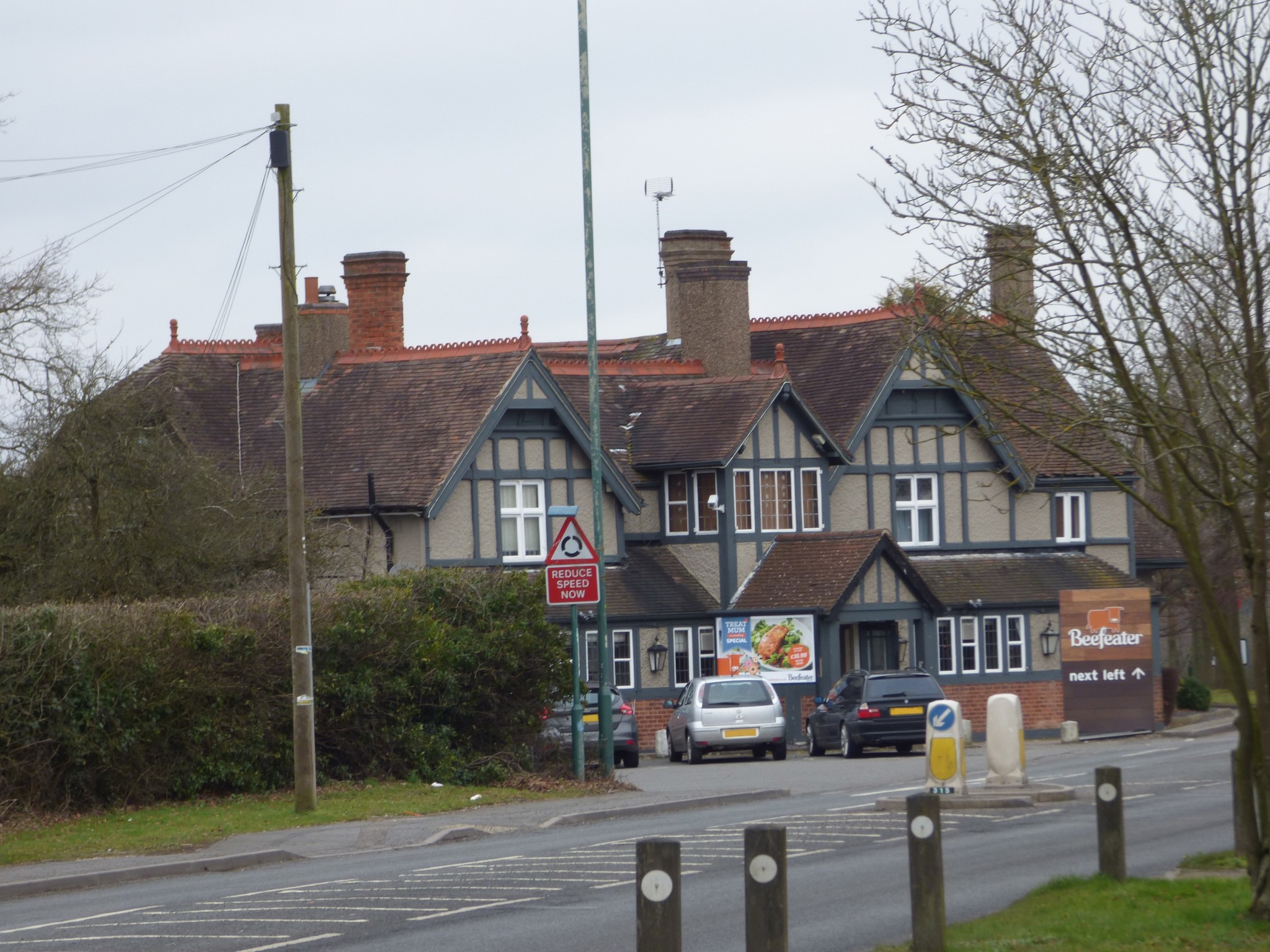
[{"xmin": 1063, "ymin": 660, "xmax": 1154, "ymax": 733}]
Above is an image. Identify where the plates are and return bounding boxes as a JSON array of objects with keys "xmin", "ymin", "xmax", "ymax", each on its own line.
[{"xmin": 746, "ymin": 617, "xmax": 813, "ymax": 671}]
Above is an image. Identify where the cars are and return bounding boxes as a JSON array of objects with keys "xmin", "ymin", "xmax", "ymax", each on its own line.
[
  {"xmin": 662, "ymin": 675, "xmax": 787, "ymax": 765},
  {"xmin": 518, "ymin": 681, "xmax": 639, "ymax": 768}
]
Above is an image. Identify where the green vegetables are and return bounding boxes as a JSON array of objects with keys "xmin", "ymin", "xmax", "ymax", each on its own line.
[{"xmin": 752, "ymin": 617, "xmax": 803, "ymax": 668}]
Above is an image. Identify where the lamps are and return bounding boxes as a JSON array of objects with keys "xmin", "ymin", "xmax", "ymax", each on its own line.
[
  {"xmin": 968, "ymin": 599, "xmax": 982, "ymax": 608},
  {"xmin": 577, "ymin": 610, "xmax": 595, "ymax": 623},
  {"xmin": 894, "ymin": 634, "xmax": 907, "ymax": 663},
  {"xmin": 1040, "ymin": 621, "xmax": 1061, "ymax": 656},
  {"xmin": 647, "ymin": 636, "xmax": 669, "ymax": 673}
]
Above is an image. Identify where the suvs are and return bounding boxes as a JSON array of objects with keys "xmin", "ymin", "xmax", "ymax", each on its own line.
[{"xmin": 805, "ymin": 666, "xmax": 947, "ymax": 759}]
